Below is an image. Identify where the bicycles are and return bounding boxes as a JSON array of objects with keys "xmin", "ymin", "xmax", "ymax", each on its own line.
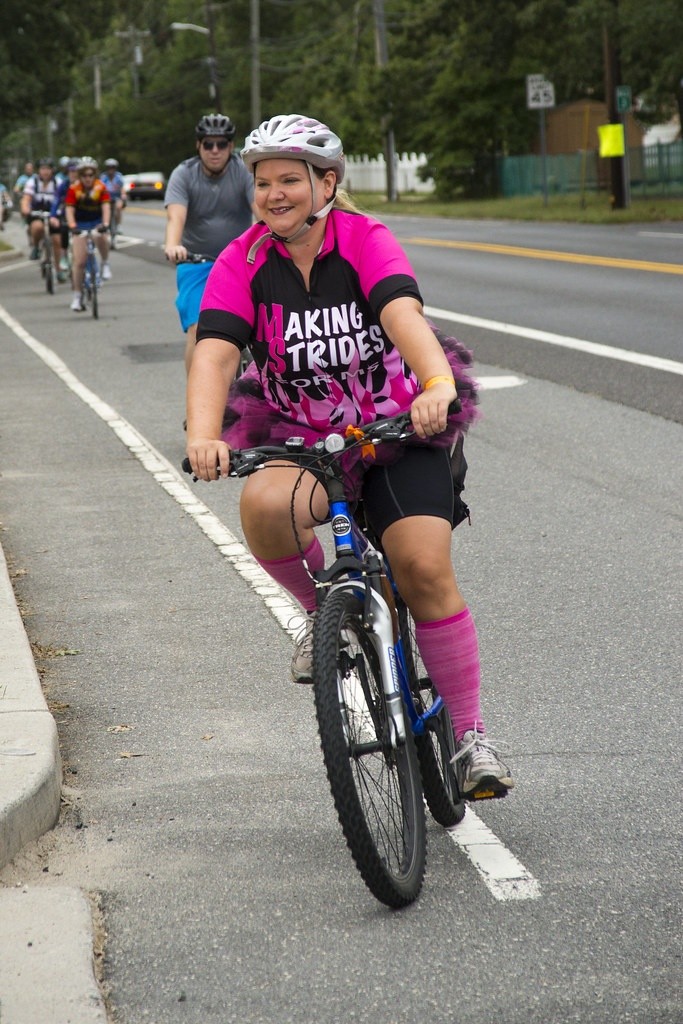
[
  {"xmin": 15, "ymin": 189, "xmax": 127, "ymax": 320},
  {"xmin": 180, "ymin": 397, "xmax": 511, "ymax": 911}
]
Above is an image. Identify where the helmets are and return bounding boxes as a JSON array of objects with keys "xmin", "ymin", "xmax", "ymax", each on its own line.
[
  {"xmin": 194, "ymin": 114, "xmax": 235, "ymax": 140},
  {"xmin": 75, "ymin": 156, "xmax": 98, "ymax": 169},
  {"xmin": 36, "ymin": 157, "xmax": 54, "ymax": 166},
  {"xmin": 58, "ymin": 156, "xmax": 70, "ymax": 166},
  {"xmin": 104, "ymin": 157, "xmax": 119, "ymax": 166},
  {"xmin": 240, "ymin": 113, "xmax": 346, "ymax": 184}
]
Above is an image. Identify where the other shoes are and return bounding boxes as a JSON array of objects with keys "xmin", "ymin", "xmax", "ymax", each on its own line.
[
  {"xmin": 30, "ymin": 250, "xmax": 41, "ymax": 261},
  {"xmin": 58, "ymin": 271, "xmax": 69, "ymax": 282},
  {"xmin": 69, "ymin": 292, "xmax": 82, "ymax": 311},
  {"xmin": 98, "ymin": 263, "xmax": 112, "ymax": 279},
  {"xmin": 60, "ymin": 259, "xmax": 69, "ymax": 271}
]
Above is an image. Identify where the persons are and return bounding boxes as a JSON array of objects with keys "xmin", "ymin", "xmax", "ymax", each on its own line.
[
  {"xmin": 163, "ymin": 113, "xmax": 263, "ymax": 376},
  {"xmin": 64, "ymin": 155, "xmax": 112, "ymax": 311},
  {"xmin": 0, "ymin": 183, "xmax": 13, "ymax": 229},
  {"xmin": 21, "ymin": 157, "xmax": 66, "ymax": 282},
  {"xmin": 100, "ymin": 158, "xmax": 127, "ymax": 231},
  {"xmin": 186, "ymin": 114, "xmax": 514, "ymax": 797},
  {"xmin": 54, "ymin": 156, "xmax": 70, "ymax": 186},
  {"xmin": 50, "ymin": 157, "xmax": 80, "ymax": 229},
  {"xmin": 14, "ymin": 160, "xmax": 37, "ymax": 192}
]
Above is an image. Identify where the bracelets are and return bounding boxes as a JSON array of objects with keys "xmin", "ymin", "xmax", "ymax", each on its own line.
[{"xmin": 425, "ymin": 376, "xmax": 455, "ymax": 389}]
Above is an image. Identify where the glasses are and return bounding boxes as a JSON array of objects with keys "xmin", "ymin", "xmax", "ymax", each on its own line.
[{"xmin": 200, "ymin": 140, "xmax": 230, "ymax": 150}]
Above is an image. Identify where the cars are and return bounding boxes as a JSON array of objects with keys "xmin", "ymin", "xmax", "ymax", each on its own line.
[{"xmin": 126, "ymin": 170, "xmax": 166, "ymax": 200}]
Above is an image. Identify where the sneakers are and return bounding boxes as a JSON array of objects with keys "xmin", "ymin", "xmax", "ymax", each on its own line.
[
  {"xmin": 448, "ymin": 722, "xmax": 516, "ymax": 794},
  {"xmin": 287, "ymin": 613, "xmax": 350, "ymax": 684}
]
